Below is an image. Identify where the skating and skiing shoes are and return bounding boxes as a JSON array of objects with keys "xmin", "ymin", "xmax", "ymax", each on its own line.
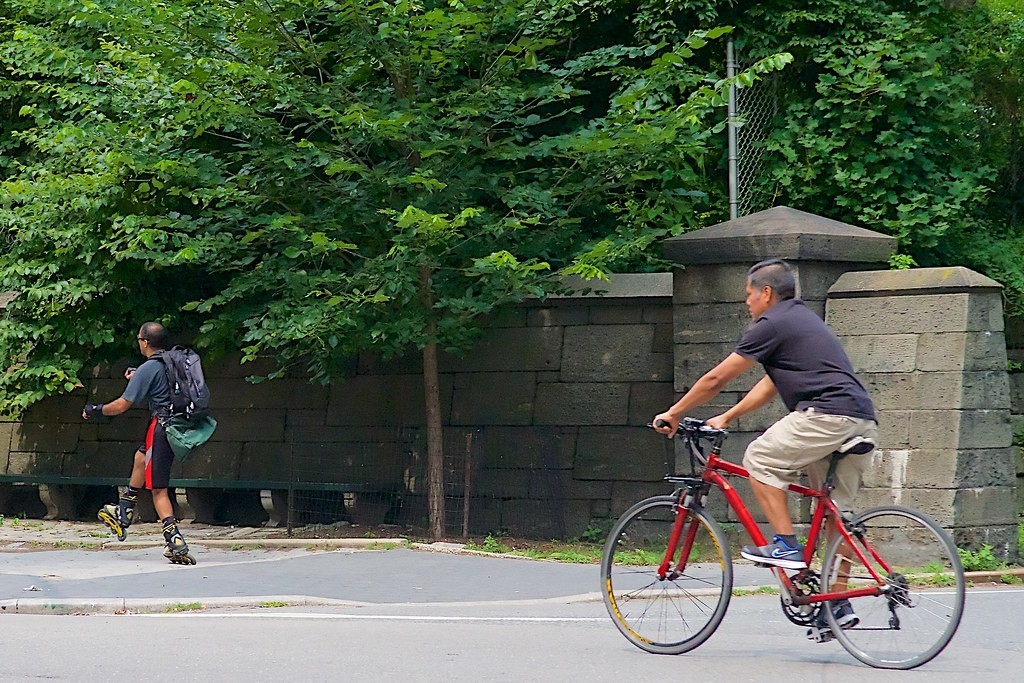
[
  {"xmin": 162, "ymin": 526, "xmax": 196, "ymax": 565},
  {"xmin": 96, "ymin": 498, "xmax": 137, "ymax": 541}
]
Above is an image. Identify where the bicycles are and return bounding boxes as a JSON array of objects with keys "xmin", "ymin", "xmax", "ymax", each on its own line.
[{"xmin": 601, "ymin": 418, "xmax": 966, "ymax": 670}]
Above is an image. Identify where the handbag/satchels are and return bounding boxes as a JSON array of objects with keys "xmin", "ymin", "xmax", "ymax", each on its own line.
[{"xmin": 162, "ymin": 413, "xmax": 218, "ymax": 463}]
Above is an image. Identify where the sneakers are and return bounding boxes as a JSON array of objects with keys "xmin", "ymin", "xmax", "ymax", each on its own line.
[
  {"xmin": 807, "ymin": 600, "xmax": 860, "ymax": 640},
  {"xmin": 741, "ymin": 535, "xmax": 807, "ymax": 570}
]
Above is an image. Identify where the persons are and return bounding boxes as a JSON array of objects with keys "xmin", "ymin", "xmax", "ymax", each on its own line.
[
  {"xmin": 81, "ymin": 322, "xmax": 197, "ymax": 558},
  {"xmin": 654, "ymin": 258, "xmax": 880, "ymax": 639}
]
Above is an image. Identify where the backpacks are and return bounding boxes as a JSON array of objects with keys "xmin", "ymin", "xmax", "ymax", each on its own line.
[{"xmin": 148, "ymin": 345, "xmax": 211, "ymax": 419}]
